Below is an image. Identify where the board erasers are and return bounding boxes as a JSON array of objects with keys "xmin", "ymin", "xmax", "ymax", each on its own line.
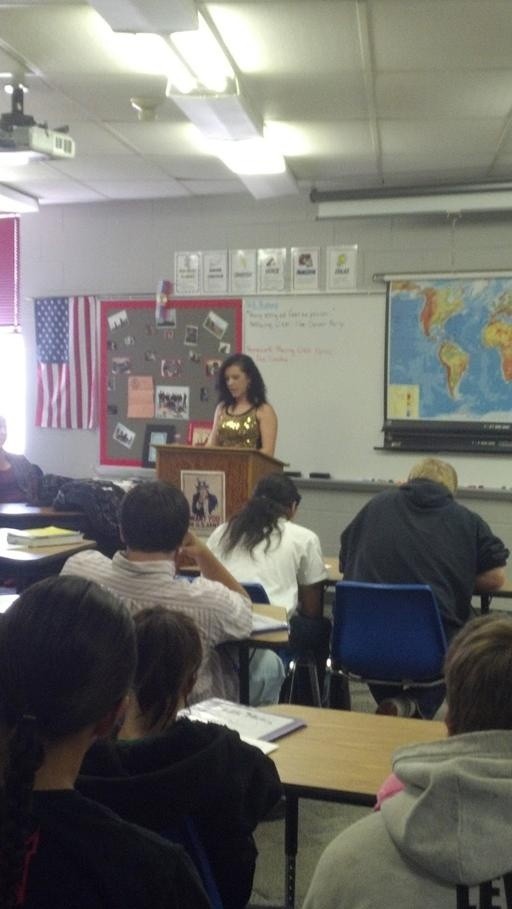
[
  {"xmin": 310, "ymin": 473, "xmax": 330, "ymax": 479},
  {"xmin": 283, "ymin": 472, "xmax": 303, "ymax": 479}
]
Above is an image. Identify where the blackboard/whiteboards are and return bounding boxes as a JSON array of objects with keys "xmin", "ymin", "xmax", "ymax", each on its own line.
[{"xmin": 238, "ymin": 290, "xmax": 512, "ymax": 499}]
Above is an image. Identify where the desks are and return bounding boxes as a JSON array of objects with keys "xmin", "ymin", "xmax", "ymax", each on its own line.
[
  {"xmin": 178, "ymin": 556, "xmax": 512, "ymax": 615},
  {"xmin": 1, "ymin": 537, "xmax": 99, "ymax": 590},
  {"xmin": 237, "ymin": 604, "xmax": 289, "ymax": 706},
  {"xmin": 0, "ymin": 504, "xmax": 88, "ymax": 524},
  {"xmin": 257, "ymin": 704, "xmax": 449, "ymax": 908}
]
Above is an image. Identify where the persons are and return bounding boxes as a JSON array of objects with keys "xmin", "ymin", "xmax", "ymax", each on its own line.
[
  {"xmin": 74, "ymin": 605, "xmax": 286, "ymax": 909},
  {"xmin": 0, "ymin": 575, "xmax": 211, "ymax": 908},
  {"xmin": 0, "ymin": 412, "xmax": 41, "ymax": 504},
  {"xmin": 204, "ymin": 354, "xmax": 277, "ymax": 456},
  {"xmin": 58, "ymin": 480, "xmax": 288, "ymax": 708},
  {"xmin": 337, "ymin": 461, "xmax": 509, "ymax": 721},
  {"xmin": 202, "ymin": 472, "xmax": 351, "ymax": 711},
  {"xmin": 298, "ymin": 616, "xmax": 512, "ymax": 908}
]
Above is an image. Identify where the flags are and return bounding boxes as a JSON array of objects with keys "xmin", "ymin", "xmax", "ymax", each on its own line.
[{"xmin": 32, "ymin": 294, "xmax": 101, "ymax": 429}]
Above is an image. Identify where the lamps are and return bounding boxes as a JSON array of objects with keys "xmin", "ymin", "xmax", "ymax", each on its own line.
[
  {"xmin": 163, "ymin": 78, "xmax": 268, "ymax": 141},
  {"xmin": 86, "ymin": 0, "xmax": 200, "ymax": 35},
  {"xmin": 234, "ymin": 155, "xmax": 301, "ymax": 200}
]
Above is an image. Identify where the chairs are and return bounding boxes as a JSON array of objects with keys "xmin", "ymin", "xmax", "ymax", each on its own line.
[
  {"xmin": 321, "ymin": 581, "xmax": 448, "ymax": 712},
  {"xmin": 184, "ymin": 576, "xmax": 320, "ymax": 710}
]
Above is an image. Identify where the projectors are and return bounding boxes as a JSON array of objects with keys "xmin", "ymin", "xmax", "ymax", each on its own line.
[{"xmin": 0, "ymin": 90, "xmax": 76, "ymax": 159}]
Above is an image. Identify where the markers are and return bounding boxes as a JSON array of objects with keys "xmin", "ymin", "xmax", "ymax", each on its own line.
[
  {"xmin": 478, "ymin": 484, "xmax": 506, "ymax": 489},
  {"xmin": 371, "ymin": 477, "xmax": 394, "ymax": 484}
]
